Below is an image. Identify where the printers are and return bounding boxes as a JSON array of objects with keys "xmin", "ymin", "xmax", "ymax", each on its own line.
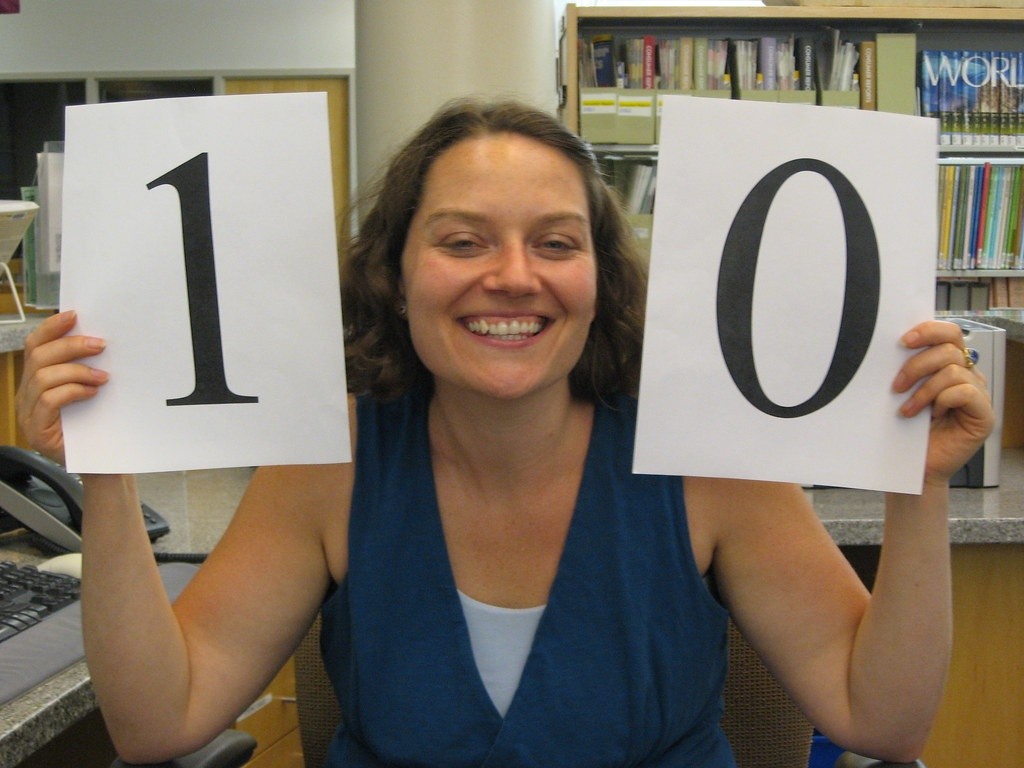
[{"xmin": 800, "ymin": 317, "xmax": 1007, "ymax": 489}]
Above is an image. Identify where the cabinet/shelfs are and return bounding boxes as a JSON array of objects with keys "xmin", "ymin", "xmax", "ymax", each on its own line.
[{"xmin": 555, "ymin": 0, "xmax": 1024, "ymax": 447}]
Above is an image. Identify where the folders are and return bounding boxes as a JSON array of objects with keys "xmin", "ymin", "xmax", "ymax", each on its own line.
[{"xmin": 936, "ymin": 277, "xmax": 1024, "ymax": 311}]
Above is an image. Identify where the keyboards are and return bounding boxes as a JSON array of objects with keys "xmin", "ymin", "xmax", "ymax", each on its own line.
[{"xmin": 0, "ymin": 558, "xmax": 81, "ymax": 643}]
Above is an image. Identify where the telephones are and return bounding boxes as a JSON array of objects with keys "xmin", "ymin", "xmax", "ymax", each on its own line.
[{"xmin": 0, "ymin": 444, "xmax": 171, "ymax": 560}]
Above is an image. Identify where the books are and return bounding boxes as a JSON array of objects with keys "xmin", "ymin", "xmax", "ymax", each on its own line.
[
  {"xmin": 0, "ymin": 198, "xmax": 39, "ymax": 276},
  {"xmin": 936, "ymin": 276, "xmax": 1024, "ymax": 311},
  {"xmin": 576, "ymin": 24, "xmax": 877, "ymax": 113},
  {"xmin": 938, "ymin": 164, "xmax": 1024, "ymax": 270},
  {"xmin": 612, "ymin": 159, "xmax": 658, "ymax": 215},
  {"xmin": 917, "ymin": 48, "xmax": 1024, "ymax": 147}
]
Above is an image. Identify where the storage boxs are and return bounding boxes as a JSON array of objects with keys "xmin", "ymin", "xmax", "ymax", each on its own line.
[
  {"xmin": 692, "ymin": 39, "xmax": 732, "ymax": 100},
  {"xmin": 726, "ymin": 38, "xmax": 778, "ymax": 103},
  {"xmin": 811, "ymin": 25, "xmax": 861, "ymax": 110},
  {"xmin": 655, "ymin": 38, "xmax": 693, "ymax": 143},
  {"xmin": 606, "ymin": 161, "xmax": 657, "ymax": 292},
  {"xmin": 577, "ymin": 35, "xmax": 617, "ymax": 143},
  {"xmin": 617, "ymin": 35, "xmax": 655, "ymax": 145},
  {"xmin": 778, "ymin": 32, "xmax": 817, "ymax": 105}
]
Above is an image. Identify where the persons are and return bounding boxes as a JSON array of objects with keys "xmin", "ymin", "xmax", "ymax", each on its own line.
[{"xmin": 13, "ymin": 93, "xmax": 996, "ymax": 768}]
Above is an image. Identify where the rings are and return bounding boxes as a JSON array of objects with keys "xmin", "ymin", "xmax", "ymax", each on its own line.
[{"xmin": 963, "ymin": 348, "xmax": 979, "ymax": 368}]
